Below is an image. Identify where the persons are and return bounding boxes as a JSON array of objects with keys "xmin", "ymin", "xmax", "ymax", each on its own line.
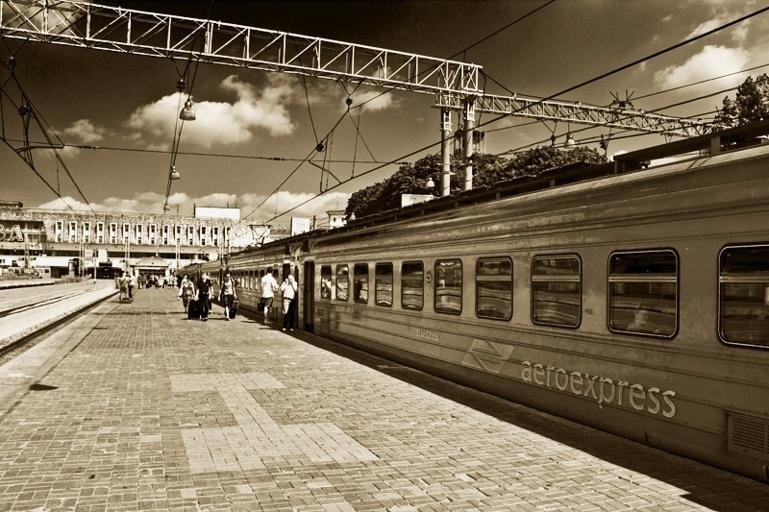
[
  {"xmin": 114, "ymin": 270, "xmax": 179, "ymax": 303},
  {"xmin": 176, "ymin": 267, "xmax": 239, "ymax": 322},
  {"xmin": 279, "ymin": 274, "xmax": 297, "ymax": 332},
  {"xmin": 258, "ymin": 265, "xmax": 278, "ymax": 326}
]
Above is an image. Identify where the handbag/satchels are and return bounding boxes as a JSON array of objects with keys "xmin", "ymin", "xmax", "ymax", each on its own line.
[{"xmin": 257, "ymin": 298, "xmax": 265, "ymax": 312}]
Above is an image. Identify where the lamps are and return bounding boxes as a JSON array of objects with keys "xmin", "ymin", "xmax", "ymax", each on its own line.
[
  {"xmin": 163, "ymin": 201, "xmax": 171, "ymax": 212},
  {"xmin": 179, "ymin": 94, "xmax": 197, "ymax": 121},
  {"xmin": 18, "ymin": 93, "xmax": 27, "ymax": 116},
  {"xmin": 563, "ymin": 120, "xmax": 577, "ymax": 149},
  {"xmin": 169, "ymin": 162, "xmax": 181, "ymax": 181},
  {"xmin": 8, "ymin": 55, "xmax": 18, "ymax": 74}
]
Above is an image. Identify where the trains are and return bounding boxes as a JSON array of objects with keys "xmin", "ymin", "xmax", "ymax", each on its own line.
[{"xmin": 175, "ymin": 121, "xmax": 769, "ymax": 487}]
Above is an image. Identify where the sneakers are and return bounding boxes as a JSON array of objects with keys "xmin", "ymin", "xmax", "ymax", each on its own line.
[
  {"xmin": 201, "ymin": 317, "xmax": 208, "ymax": 321},
  {"xmin": 282, "ymin": 328, "xmax": 294, "ymax": 331}
]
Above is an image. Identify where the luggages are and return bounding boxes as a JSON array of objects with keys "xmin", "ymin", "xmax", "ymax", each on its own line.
[
  {"xmin": 229, "ymin": 298, "xmax": 239, "ymax": 319},
  {"xmin": 188, "ymin": 300, "xmax": 202, "ymax": 319}
]
